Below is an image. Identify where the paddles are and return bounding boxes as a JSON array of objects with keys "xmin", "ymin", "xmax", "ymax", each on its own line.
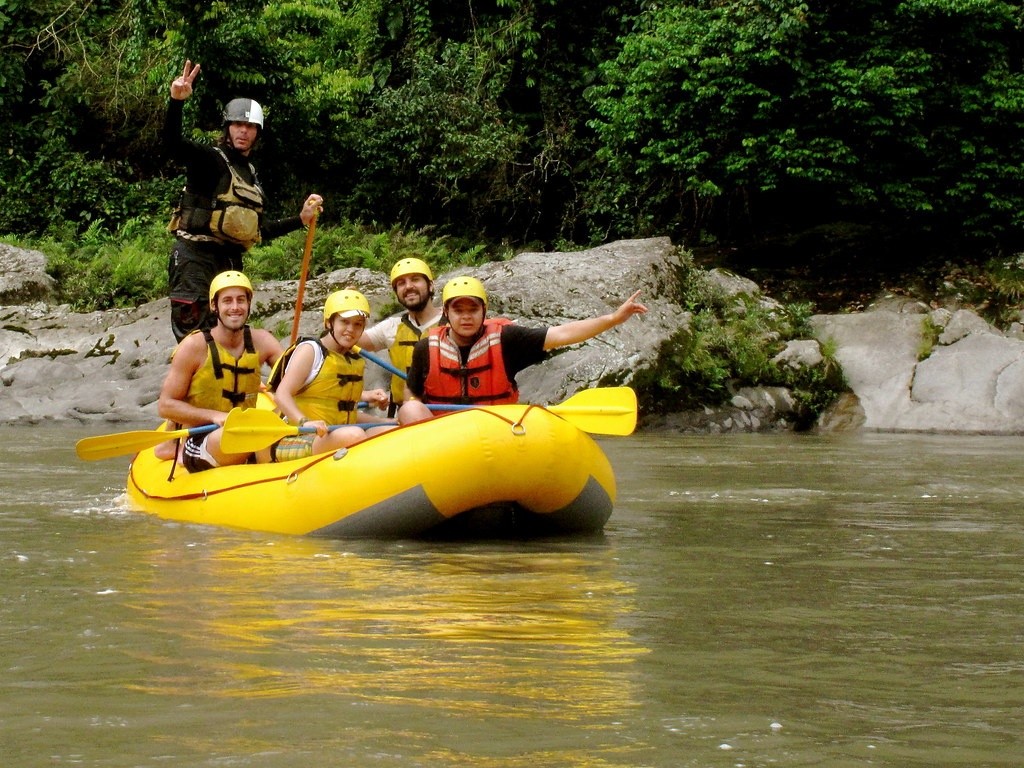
[
  {"xmin": 350, "ymin": 342, "xmax": 406, "ymax": 386},
  {"xmin": 290, "ymin": 201, "xmax": 325, "ymax": 346},
  {"xmin": 410, "ymin": 385, "xmax": 639, "ymax": 437},
  {"xmin": 220, "ymin": 405, "xmax": 402, "ymax": 456},
  {"xmin": 74, "ymin": 399, "xmax": 372, "ymax": 462}
]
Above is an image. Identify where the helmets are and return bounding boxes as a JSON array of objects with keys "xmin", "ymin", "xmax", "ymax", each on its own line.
[
  {"xmin": 443, "ymin": 277, "xmax": 488, "ymax": 311},
  {"xmin": 209, "ymin": 270, "xmax": 253, "ymax": 313},
  {"xmin": 390, "ymin": 258, "xmax": 433, "ymax": 288},
  {"xmin": 324, "ymin": 290, "xmax": 371, "ymax": 331},
  {"xmin": 221, "ymin": 97, "xmax": 264, "ymax": 130}
]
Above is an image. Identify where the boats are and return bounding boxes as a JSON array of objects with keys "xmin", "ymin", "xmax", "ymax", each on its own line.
[{"xmin": 125, "ymin": 378, "xmax": 618, "ymax": 541}]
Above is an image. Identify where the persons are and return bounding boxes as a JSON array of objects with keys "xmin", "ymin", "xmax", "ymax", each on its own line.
[
  {"xmin": 396, "ymin": 274, "xmax": 647, "ymax": 425},
  {"xmin": 154, "ymin": 58, "xmax": 324, "ymax": 461},
  {"xmin": 263, "ymin": 258, "xmax": 444, "ymax": 461},
  {"xmin": 159, "ymin": 270, "xmax": 285, "ymax": 473}
]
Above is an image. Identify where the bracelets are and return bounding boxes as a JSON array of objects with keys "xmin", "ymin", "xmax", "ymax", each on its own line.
[{"xmin": 300, "ymin": 417, "xmax": 310, "ymax": 426}]
